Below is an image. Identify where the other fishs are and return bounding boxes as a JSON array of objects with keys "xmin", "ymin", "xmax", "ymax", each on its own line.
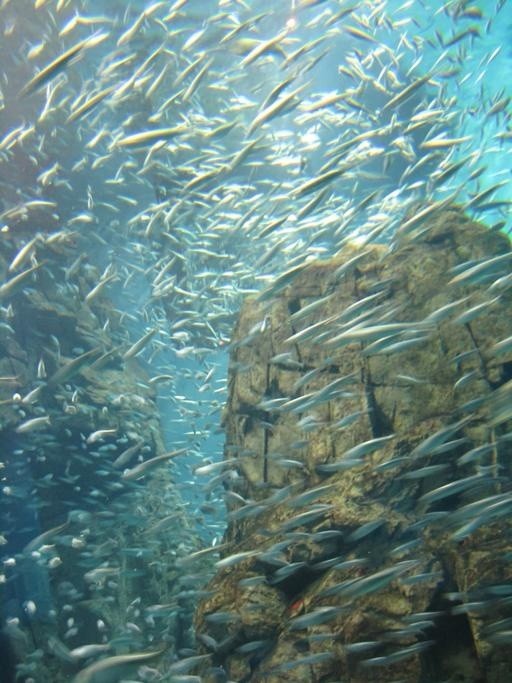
[{"xmin": 0, "ymin": 0, "xmax": 512, "ymax": 683}]
